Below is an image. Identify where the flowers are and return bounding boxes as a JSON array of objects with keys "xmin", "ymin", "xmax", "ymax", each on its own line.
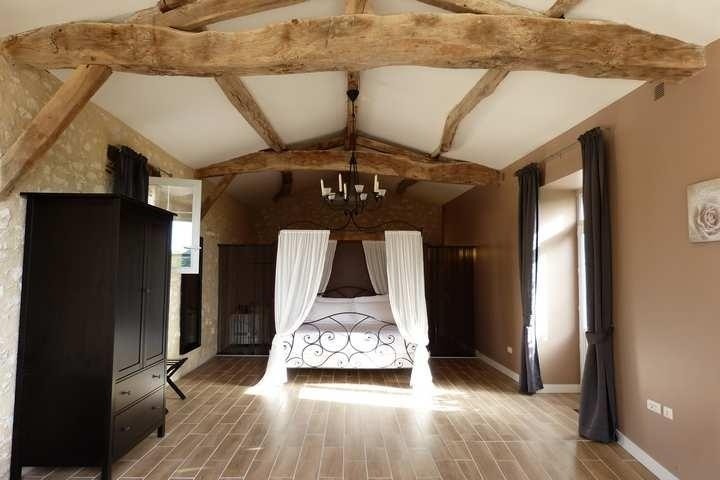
[{"xmin": 692, "ymin": 200, "xmax": 720, "ymax": 239}]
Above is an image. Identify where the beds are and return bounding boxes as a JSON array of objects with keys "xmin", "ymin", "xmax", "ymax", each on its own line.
[{"xmin": 257, "ymin": 229, "xmax": 437, "ymax": 394}]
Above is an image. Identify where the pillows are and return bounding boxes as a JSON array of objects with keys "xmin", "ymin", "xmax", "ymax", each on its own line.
[{"xmin": 304, "ymin": 293, "xmax": 395, "ymax": 323}]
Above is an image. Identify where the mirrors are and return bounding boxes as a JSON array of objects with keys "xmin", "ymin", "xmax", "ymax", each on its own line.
[{"xmin": 179, "ymin": 235, "xmax": 204, "ymax": 355}]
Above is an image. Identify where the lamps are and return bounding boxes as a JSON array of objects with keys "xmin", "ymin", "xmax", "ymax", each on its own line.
[{"xmin": 317, "ymin": 89, "xmax": 389, "ymax": 214}]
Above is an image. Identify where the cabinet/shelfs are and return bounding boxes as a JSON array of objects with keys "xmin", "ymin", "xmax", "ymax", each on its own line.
[
  {"xmin": 427, "ymin": 244, "xmax": 478, "ymax": 357},
  {"xmin": 8, "ymin": 186, "xmax": 177, "ymax": 479},
  {"xmin": 218, "ymin": 242, "xmax": 277, "ymax": 356}
]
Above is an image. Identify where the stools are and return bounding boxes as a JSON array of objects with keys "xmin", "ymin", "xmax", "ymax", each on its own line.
[{"xmin": 163, "ymin": 356, "xmax": 188, "ymax": 400}]
